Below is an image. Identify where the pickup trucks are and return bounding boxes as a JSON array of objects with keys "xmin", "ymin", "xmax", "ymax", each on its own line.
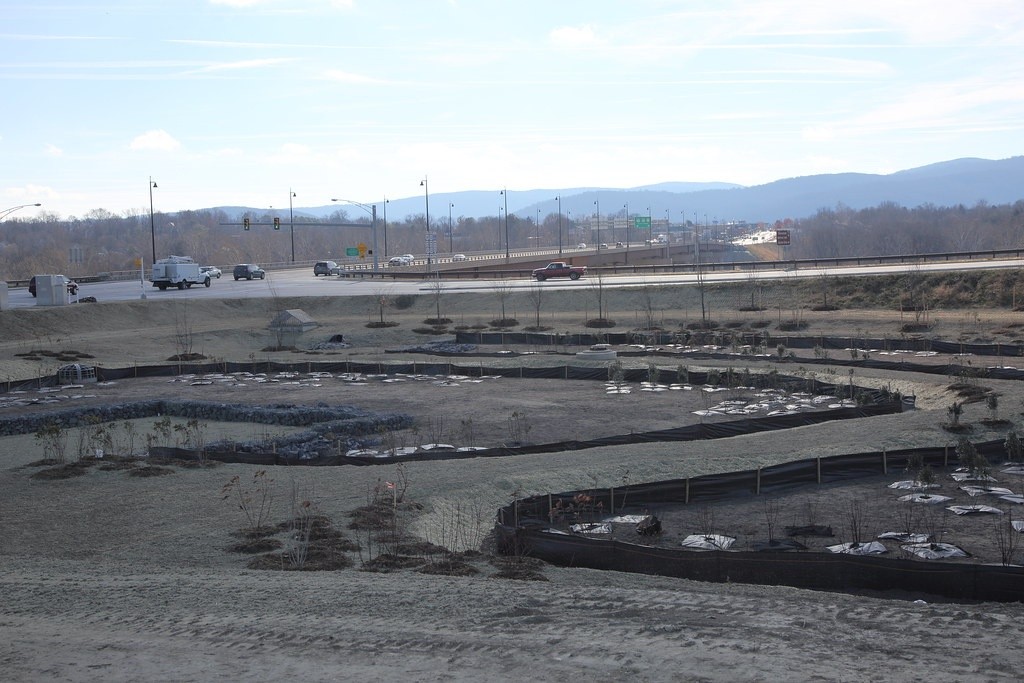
[{"xmin": 531, "ymin": 261, "xmax": 589, "ymax": 280}]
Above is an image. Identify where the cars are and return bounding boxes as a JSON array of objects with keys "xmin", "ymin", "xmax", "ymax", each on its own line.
[
  {"xmin": 616, "ymin": 242, "xmax": 623, "ymax": 247},
  {"xmin": 600, "ymin": 244, "xmax": 608, "ymax": 249},
  {"xmin": 402, "ymin": 254, "xmax": 414, "ymax": 262},
  {"xmin": 578, "ymin": 243, "xmax": 586, "ymax": 249},
  {"xmin": 201, "ymin": 266, "xmax": 222, "ymax": 279},
  {"xmin": 452, "ymin": 253, "xmax": 465, "ymax": 261},
  {"xmin": 389, "ymin": 257, "xmax": 409, "ymax": 267}
]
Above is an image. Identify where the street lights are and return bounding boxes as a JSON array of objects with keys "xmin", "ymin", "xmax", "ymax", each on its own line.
[
  {"xmin": 665, "ymin": 207, "xmax": 669, "ymax": 245},
  {"xmin": 593, "ymin": 198, "xmax": 601, "ymax": 250},
  {"xmin": 680, "ymin": 210, "xmax": 686, "ymax": 244},
  {"xmin": 712, "ymin": 215, "xmax": 718, "ymax": 242},
  {"xmin": 289, "ymin": 188, "xmax": 297, "ymax": 262},
  {"xmin": 498, "ymin": 204, "xmax": 503, "ymax": 252},
  {"xmin": 566, "ymin": 209, "xmax": 571, "ymax": 245},
  {"xmin": 703, "ymin": 213, "xmax": 708, "ymax": 241},
  {"xmin": 555, "ymin": 194, "xmax": 562, "ymax": 253},
  {"xmin": 693, "ymin": 210, "xmax": 698, "ymax": 243},
  {"xmin": 500, "ymin": 188, "xmax": 509, "ymax": 259},
  {"xmin": 536, "ymin": 208, "xmax": 541, "ymax": 251},
  {"xmin": 449, "ymin": 202, "xmax": 455, "ymax": 253},
  {"xmin": 149, "ymin": 175, "xmax": 160, "ymax": 267},
  {"xmin": 623, "ymin": 203, "xmax": 630, "ymax": 247},
  {"xmin": 647, "ymin": 201, "xmax": 651, "ymax": 249},
  {"xmin": 419, "ymin": 175, "xmax": 430, "ymax": 269},
  {"xmin": 384, "ymin": 194, "xmax": 390, "ymax": 259},
  {"xmin": 330, "ymin": 198, "xmax": 379, "ymax": 278}
]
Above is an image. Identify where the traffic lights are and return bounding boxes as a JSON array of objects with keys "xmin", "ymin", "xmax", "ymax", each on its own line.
[
  {"xmin": 274, "ymin": 217, "xmax": 280, "ymax": 230},
  {"xmin": 243, "ymin": 218, "xmax": 249, "ymax": 231}
]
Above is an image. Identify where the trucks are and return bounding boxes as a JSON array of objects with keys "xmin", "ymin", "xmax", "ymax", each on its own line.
[{"xmin": 150, "ymin": 255, "xmax": 211, "ymax": 289}]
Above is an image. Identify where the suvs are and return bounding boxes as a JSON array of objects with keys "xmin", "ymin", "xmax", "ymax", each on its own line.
[
  {"xmin": 313, "ymin": 260, "xmax": 340, "ymax": 275},
  {"xmin": 28, "ymin": 274, "xmax": 79, "ymax": 296},
  {"xmin": 232, "ymin": 264, "xmax": 266, "ymax": 280}
]
